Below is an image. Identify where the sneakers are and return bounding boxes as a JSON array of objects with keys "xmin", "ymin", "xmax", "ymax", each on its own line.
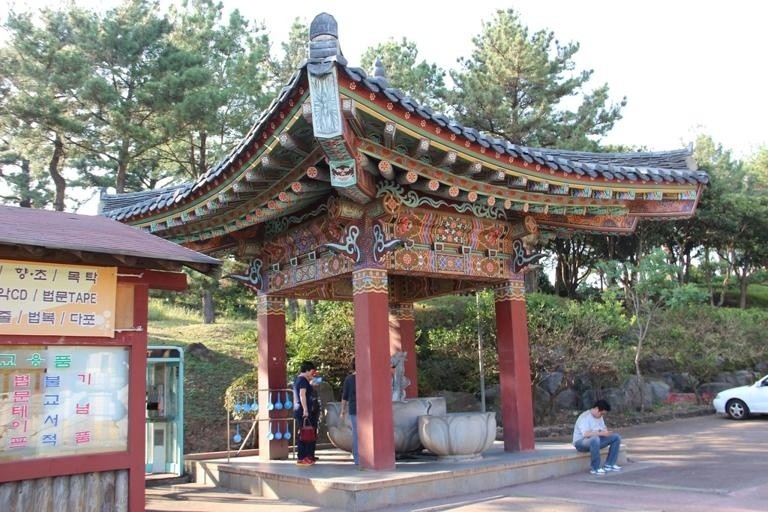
[
  {"xmin": 590, "ymin": 465, "xmax": 624, "ymax": 474},
  {"xmin": 297, "ymin": 457, "xmax": 319, "ymax": 465}
]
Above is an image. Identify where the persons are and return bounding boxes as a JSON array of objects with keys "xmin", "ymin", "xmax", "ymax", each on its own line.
[
  {"xmin": 340, "ymin": 357, "xmax": 359, "ymax": 465},
  {"xmin": 573, "ymin": 400, "xmax": 622, "ymax": 474},
  {"xmin": 293, "ymin": 361, "xmax": 319, "ymax": 466}
]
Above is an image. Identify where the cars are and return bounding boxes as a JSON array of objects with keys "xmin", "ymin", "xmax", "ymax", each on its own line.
[{"xmin": 712, "ymin": 375, "xmax": 768, "ymax": 420}]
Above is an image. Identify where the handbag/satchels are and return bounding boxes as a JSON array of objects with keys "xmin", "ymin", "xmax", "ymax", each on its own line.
[{"xmin": 300, "ymin": 418, "xmax": 317, "ymax": 442}]
